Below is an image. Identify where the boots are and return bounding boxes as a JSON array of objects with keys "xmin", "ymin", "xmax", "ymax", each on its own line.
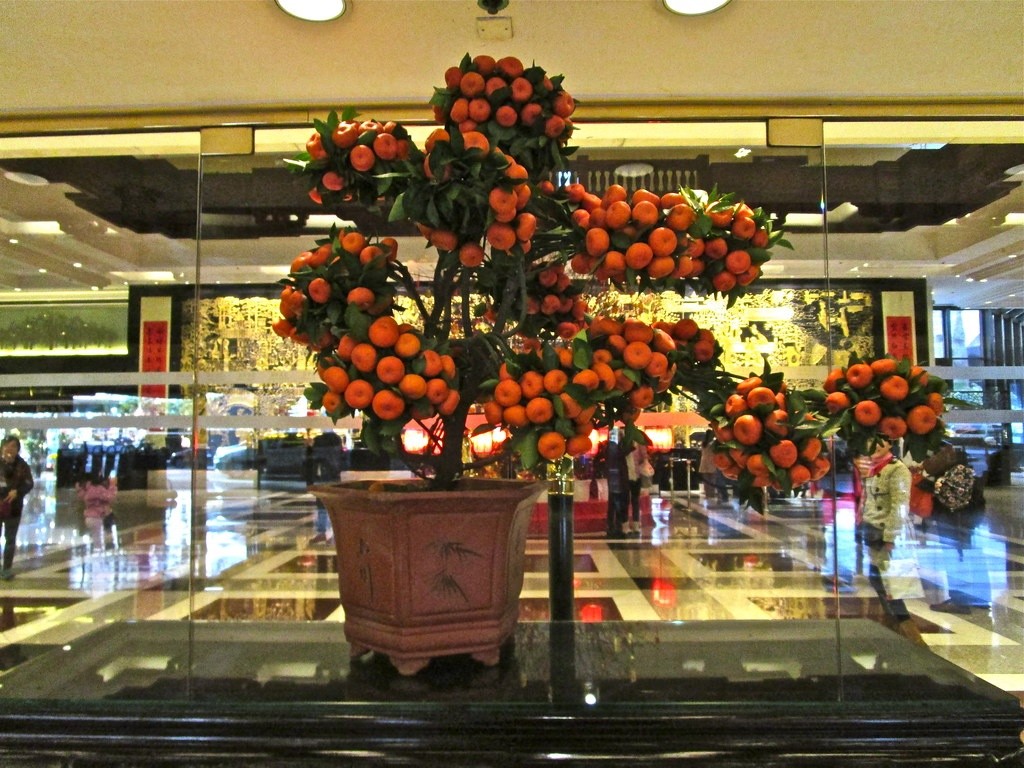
[{"xmin": 898, "ymin": 619, "xmax": 931, "ymax": 651}]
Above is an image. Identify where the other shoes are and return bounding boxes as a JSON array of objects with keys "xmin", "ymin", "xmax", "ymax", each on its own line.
[
  {"xmin": 2, "ymin": 568, "xmax": 16, "ymax": 581},
  {"xmin": 633, "ymin": 522, "xmax": 640, "ymax": 533},
  {"xmin": 622, "ymin": 521, "xmax": 632, "ymax": 534},
  {"xmin": 309, "ymin": 533, "xmax": 336, "ymax": 547}
]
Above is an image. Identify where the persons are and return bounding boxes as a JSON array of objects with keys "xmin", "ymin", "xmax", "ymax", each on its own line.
[
  {"xmin": 307, "ymin": 427, "xmax": 344, "ymax": 544},
  {"xmin": 0, "ymin": 436, "xmax": 34, "ymax": 578},
  {"xmin": 922, "ymin": 428, "xmax": 992, "ymax": 614},
  {"xmin": 605, "ymin": 426, "xmax": 649, "ymax": 537},
  {"xmin": 862, "ymin": 433, "xmax": 912, "ymax": 619}
]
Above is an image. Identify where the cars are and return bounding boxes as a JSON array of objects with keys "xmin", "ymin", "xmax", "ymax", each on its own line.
[
  {"xmin": 171, "ymin": 447, "xmax": 212, "ymax": 468},
  {"xmin": 951, "ymin": 422, "xmax": 1004, "ymax": 438},
  {"xmin": 213, "ymin": 437, "xmax": 302, "ymax": 471}
]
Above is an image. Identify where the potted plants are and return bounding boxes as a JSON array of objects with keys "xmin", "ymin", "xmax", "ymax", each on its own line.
[{"xmin": 267, "ymin": 57, "xmax": 955, "ymax": 675}]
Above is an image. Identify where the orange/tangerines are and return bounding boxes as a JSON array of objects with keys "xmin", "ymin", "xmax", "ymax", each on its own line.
[{"xmin": 271, "ymin": 56, "xmax": 947, "ymax": 493}]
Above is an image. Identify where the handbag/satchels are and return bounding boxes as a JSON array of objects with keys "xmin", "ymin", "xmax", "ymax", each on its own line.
[
  {"xmin": 908, "ymin": 473, "xmax": 934, "ymax": 519},
  {"xmin": 637, "ymin": 459, "xmax": 655, "ymax": 478},
  {"xmin": 855, "ymin": 521, "xmax": 886, "ymax": 552}
]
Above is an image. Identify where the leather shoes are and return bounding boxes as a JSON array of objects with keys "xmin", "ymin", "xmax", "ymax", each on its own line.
[
  {"xmin": 968, "ymin": 595, "xmax": 992, "ymax": 610},
  {"xmin": 929, "ymin": 599, "xmax": 970, "ymax": 615}
]
